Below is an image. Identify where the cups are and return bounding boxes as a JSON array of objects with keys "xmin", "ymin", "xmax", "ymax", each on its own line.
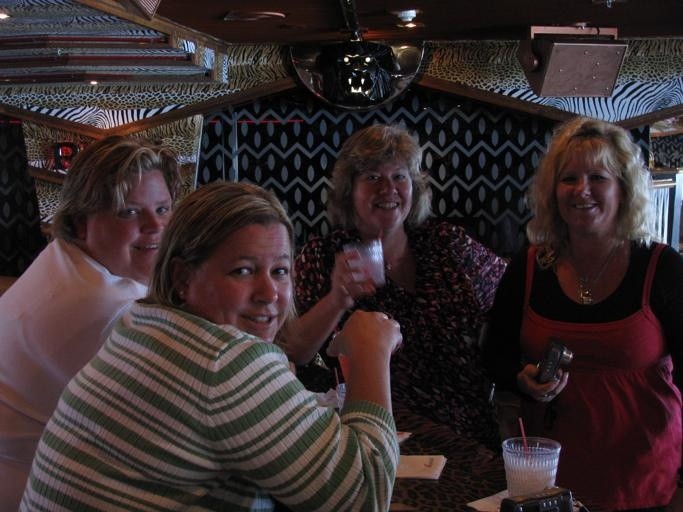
[
  {"xmin": 501, "ymin": 437, "xmax": 561, "ymax": 498},
  {"xmin": 343, "ymin": 239, "xmax": 385, "ymax": 288},
  {"xmin": 335, "ymin": 384, "xmax": 346, "ymax": 414},
  {"xmin": 332, "ymin": 331, "xmax": 349, "ymax": 383}
]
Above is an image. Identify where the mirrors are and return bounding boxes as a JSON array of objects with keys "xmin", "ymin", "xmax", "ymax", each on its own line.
[{"xmin": 277, "ymin": 1, "xmax": 432, "ymax": 110}]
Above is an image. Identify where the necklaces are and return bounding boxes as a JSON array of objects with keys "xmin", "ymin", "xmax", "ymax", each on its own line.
[
  {"xmin": 568, "ymin": 242, "xmax": 621, "ymax": 304},
  {"xmin": 383, "ymin": 257, "xmax": 396, "ymax": 274}
]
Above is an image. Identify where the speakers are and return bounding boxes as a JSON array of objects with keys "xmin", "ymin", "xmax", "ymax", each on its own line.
[{"xmin": 516, "ymin": 30, "xmax": 628, "ymax": 96}]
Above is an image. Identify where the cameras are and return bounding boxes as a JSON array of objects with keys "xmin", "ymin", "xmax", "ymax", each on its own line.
[
  {"xmin": 537, "ymin": 337, "xmax": 574, "ymax": 383},
  {"xmin": 500, "ymin": 487, "xmax": 573, "ymax": 512}
]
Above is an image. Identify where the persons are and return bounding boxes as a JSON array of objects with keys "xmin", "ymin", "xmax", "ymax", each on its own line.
[
  {"xmin": 1, "ymin": 136, "xmax": 180, "ymax": 512},
  {"xmin": 290, "ymin": 124, "xmax": 509, "ymax": 449},
  {"xmin": 19, "ymin": 181, "xmax": 404, "ymax": 512},
  {"xmin": 477, "ymin": 118, "xmax": 682, "ymax": 511}
]
